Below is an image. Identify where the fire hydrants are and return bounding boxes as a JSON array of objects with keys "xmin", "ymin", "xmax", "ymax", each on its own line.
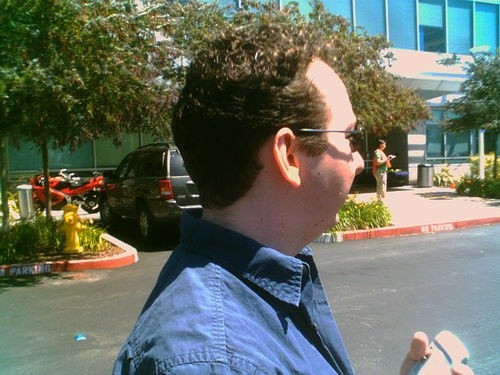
[{"xmin": 58, "ymin": 200, "xmax": 85, "ymax": 260}]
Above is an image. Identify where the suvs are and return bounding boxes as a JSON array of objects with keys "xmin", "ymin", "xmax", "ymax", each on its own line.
[{"xmin": 99, "ymin": 142, "xmax": 204, "ymax": 245}]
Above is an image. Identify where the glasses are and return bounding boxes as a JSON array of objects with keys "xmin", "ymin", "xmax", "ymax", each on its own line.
[{"xmin": 291, "ymin": 123, "xmax": 364, "ymax": 152}]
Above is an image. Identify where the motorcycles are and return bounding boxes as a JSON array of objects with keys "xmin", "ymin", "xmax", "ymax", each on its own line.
[{"xmin": 26, "ymin": 166, "xmax": 109, "ymax": 214}]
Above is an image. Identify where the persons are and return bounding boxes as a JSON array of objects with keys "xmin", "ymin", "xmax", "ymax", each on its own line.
[
  {"xmin": 372, "ymin": 139, "xmax": 392, "ymax": 202},
  {"xmin": 113, "ymin": 31, "xmax": 475, "ymax": 375}
]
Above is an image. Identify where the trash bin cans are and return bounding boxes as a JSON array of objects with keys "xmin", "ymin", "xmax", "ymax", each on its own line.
[
  {"xmin": 15, "ymin": 184, "xmax": 35, "ymax": 219},
  {"xmin": 417, "ymin": 163, "xmax": 435, "ymax": 188}
]
